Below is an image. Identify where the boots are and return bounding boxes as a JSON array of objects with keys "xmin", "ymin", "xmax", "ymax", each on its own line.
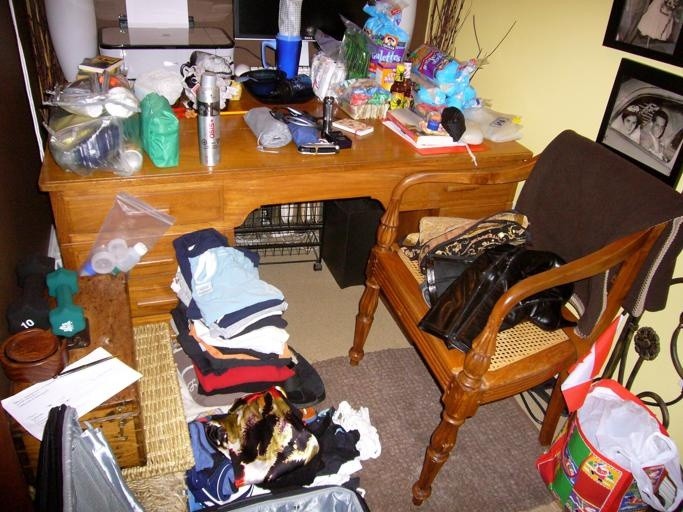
[{"xmin": 417, "ymin": 244, "xmax": 577, "ymax": 356}]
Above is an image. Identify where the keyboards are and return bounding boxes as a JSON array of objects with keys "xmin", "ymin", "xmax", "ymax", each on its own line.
[{"xmin": 251, "ymin": 66, "xmax": 310, "ymax": 77}]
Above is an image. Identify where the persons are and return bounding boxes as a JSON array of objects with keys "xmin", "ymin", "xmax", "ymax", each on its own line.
[
  {"xmin": 77, "ymin": 55, "xmax": 124, "ymax": 80},
  {"xmin": 622, "ymin": 109, "xmax": 669, "ymax": 162}
]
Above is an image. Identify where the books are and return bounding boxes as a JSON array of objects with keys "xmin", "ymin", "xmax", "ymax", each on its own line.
[
  {"xmin": 380, "ymin": 108, "xmax": 489, "ymax": 156},
  {"xmin": 331, "ymin": 117, "xmax": 375, "ymax": 136}
]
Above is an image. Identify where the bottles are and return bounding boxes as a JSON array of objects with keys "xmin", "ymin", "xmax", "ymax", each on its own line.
[
  {"xmin": 197, "ymin": 72, "xmax": 221, "ymax": 167},
  {"xmin": 90, "ymin": 238, "xmax": 148, "ymax": 274},
  {"xmin": 388, "ymin": 60, "xmax": 415, "ymax": 111}
]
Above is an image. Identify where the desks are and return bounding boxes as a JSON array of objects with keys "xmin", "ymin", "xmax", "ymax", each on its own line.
[{"xmin": 33, "ymin": 91, "xmax": 536, "ymax": 344}]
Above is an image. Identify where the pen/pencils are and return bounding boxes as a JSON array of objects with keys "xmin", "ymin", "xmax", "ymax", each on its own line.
[
  {"xmin": 220, "ymin": 111, "xmax": 247, "ymax": 115},
  {"xmin": 59, "ymin": 354, "xmax": 122, "ymax": 377}
]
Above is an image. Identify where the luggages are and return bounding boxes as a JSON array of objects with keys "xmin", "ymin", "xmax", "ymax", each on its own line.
[{"xmin": 34, "ymin": 405, "xmax": 371, "ymax": 512}]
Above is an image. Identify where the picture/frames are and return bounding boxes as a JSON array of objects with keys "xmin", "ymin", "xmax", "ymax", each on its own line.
[{"xmin": 595, "ymin": 2, "xmax": 683, "ymax": 196}]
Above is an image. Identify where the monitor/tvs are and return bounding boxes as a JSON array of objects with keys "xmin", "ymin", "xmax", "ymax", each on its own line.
[{"xmin": 233, "ymin": 0, "xmax": 375, "ymax": 42}]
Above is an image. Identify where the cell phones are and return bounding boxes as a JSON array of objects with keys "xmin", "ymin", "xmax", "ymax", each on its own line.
[{"xmin": 324, "ymin": 131, "xmax": 352, "ymax": 149}]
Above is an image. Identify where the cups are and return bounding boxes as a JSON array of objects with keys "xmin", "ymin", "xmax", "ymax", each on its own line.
[{"xmin": 261, "ymin": 33, "xmax": 302, "ymax": 79}]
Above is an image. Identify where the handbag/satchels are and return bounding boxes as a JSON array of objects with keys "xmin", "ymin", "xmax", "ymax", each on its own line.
[{"xmin": 536, "ymin": 379, "xmax": 677, "ymax": 512}]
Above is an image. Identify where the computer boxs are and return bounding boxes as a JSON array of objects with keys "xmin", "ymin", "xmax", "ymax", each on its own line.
[{"xmin": 319, "ymin": 197, "xmax": 386, "ymax": 289}]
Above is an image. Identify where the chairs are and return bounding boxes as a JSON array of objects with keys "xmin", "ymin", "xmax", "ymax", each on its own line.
[{"xmin": 345, "ymin": 127, "xmax": 683, "ymax": 505}]
